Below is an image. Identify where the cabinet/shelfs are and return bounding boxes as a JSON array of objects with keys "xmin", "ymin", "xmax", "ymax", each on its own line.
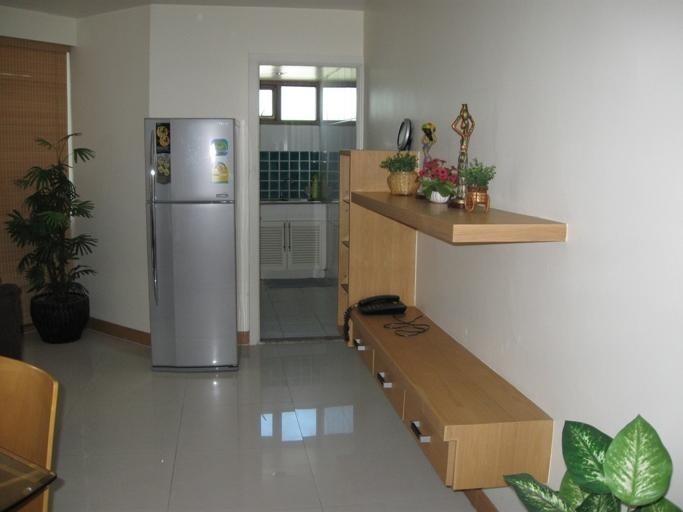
[
  {"xmin": 335, "ymin": 148, "xmax": 568, "ymax": 493},
  {"xmin": 257, "ymin": 203, "xmax": 329, "ymax": 279}
]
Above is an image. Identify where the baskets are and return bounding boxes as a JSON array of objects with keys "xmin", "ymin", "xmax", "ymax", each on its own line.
[{"xmin": 386, "ymin": 171, "xmax": 421, "ymax": 196}]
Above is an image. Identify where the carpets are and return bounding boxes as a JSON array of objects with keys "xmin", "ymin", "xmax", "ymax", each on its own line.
[{"xmin": 262, "ymin": 277, "xmax": 335, "ymax": 288}]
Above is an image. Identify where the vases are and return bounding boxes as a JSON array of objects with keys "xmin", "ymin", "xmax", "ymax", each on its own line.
[{"xmin": 425, "ymin": 188, "xmax": 450, "ymax": 204}]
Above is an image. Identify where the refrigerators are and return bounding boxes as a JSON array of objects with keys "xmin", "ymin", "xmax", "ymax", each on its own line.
[{"xmin": 140, "ymin": 117, "xmax": 240, "ymax": 374}]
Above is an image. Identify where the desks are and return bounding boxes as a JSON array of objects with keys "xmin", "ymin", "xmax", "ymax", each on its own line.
[{"xmin": 0, "ymin": 444, "xmax": 56, "ymax": 511}]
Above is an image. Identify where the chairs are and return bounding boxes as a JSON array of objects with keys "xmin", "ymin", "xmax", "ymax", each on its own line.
[{"xmin": 0, "ymin": 354, "xmax": 60, "ymax": 511}]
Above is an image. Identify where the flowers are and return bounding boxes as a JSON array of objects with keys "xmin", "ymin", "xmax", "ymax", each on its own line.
[{"xmin": 412, "ymin": 159, "xmax": 460, "ymax": 199}]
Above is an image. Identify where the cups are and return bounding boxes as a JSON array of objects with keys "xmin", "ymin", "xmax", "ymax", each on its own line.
[{"xmin": 303, "ymin": 184, "xmax": 311, "ymax": 198}]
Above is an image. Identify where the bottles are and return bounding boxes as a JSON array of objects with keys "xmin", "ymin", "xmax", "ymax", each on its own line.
[{"xmin": 310, "ymin": 175, "xmax": 319, "ymax": 200}]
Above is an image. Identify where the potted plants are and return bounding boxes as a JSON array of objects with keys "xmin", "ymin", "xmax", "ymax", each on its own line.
[
  {"xmin": 0, "ymin": 129, "xmax": 103, "ymax": 344},
  {"xmin": 379, "ymin": 152, "xmax": 419, "ymax": 198},
  {"xmin": 457, "ymin": 156, "xmax": 497, "ymax": 216}
]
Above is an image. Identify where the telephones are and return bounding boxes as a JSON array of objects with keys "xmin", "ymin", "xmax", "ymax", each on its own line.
[{"xmin": 358, "ymin": 295, "xmax": 406, "ymax": 315}]
